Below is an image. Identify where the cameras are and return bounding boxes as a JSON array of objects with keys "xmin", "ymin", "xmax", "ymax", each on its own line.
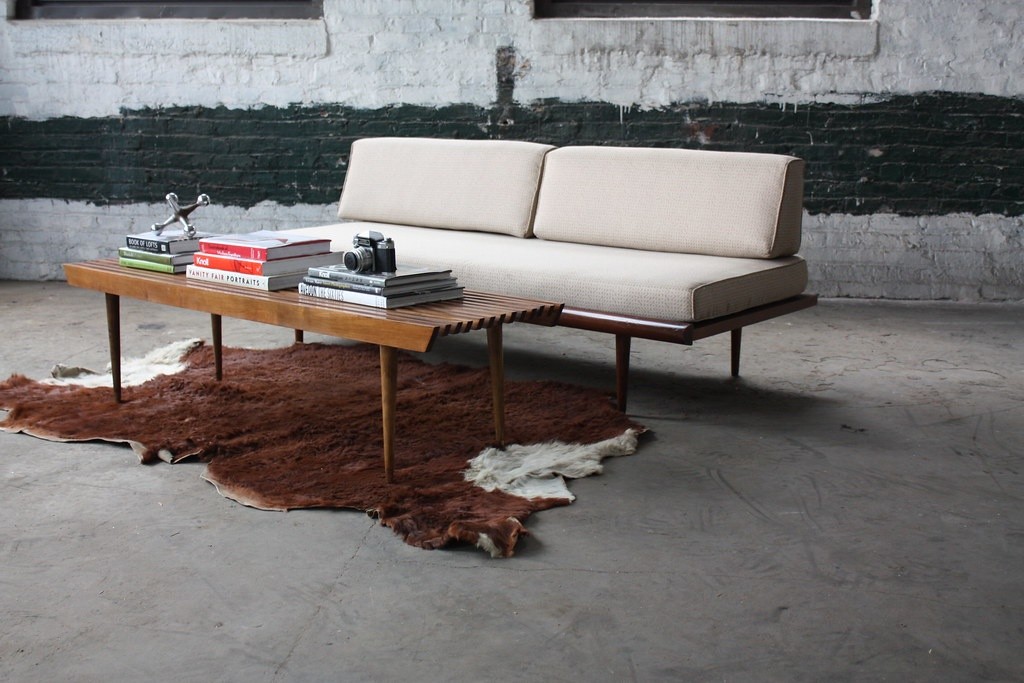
[{"xmin": 342, "ymin": 231, "xmax": 398, "ymax": 277}]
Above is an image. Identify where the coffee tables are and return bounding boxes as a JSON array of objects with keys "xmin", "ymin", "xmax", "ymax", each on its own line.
[{"xmin": 64, "ymin": 257, "xmax": 564, "ymax": 484}]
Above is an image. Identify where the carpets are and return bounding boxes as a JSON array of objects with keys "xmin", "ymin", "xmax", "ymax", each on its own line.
[{"xmin": 1, "ymin": 338, "xmax": 650, "ymax": 558}]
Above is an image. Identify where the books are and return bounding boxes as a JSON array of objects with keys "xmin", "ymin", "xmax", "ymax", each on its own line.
[
  {"xmin": 309, "ymin": 262, "xmax": 452, "ymax": 289},
  {"xmin": 119, "ymin": 247, "xmax": 195, "ymax": 265},
  {"xmin": 120, "ymin": 259, "xmax": 187, "ymax": 274},
  {"xmin": 193, "ymin": 251, "xmax": 345, "ymax": 275},
  {"xmin": 298, "ymin": 283, "xmax": 467, "ymax": 311},
  {"xmin": 304, "ymin": 276, "xmax": 458, "ymax": 297},
  {"xmin": 187, "ymin": 266, "xmax": 306, "ymax": 291},
  {"xmin": 198, "ymin": 230, "xmax": 333, "ymax": 261},
  {"xmin": 126, "ymin": 228, "xmax": 221, "ymax": 255}
]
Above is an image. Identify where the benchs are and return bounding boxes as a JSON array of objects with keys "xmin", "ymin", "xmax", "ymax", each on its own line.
[{"xmin": 278, "ymin": 136, "xmax": 818, "ymax": 413}]
